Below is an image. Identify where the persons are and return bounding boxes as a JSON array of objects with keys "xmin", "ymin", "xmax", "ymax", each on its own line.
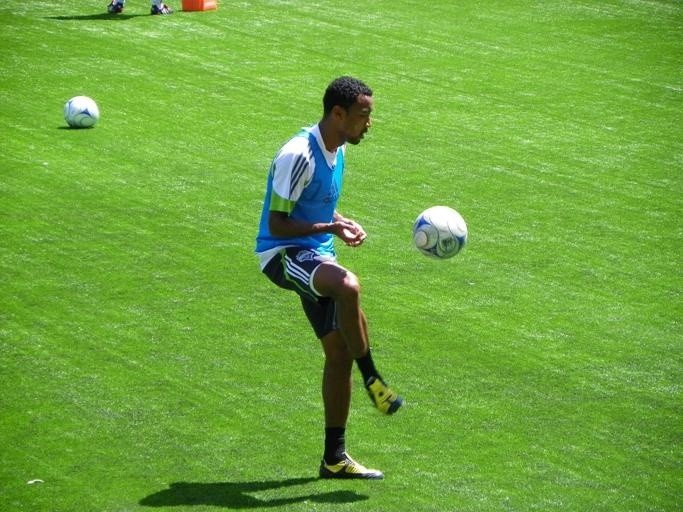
[
  {"xmin": 255, "ymin": 76, "xmax": 402, "ymax": 481},
  {"xmin": 107, "ymin": 0, "xmax": 173, "ymax": 15}
]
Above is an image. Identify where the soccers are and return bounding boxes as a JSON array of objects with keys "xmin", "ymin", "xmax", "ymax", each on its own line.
[
  {"xmin": 64, "ymin": 96, "xmax": 98, "ymax": 128},
  {"xmin": 413, "ymin": 206, "xmax": 467, "ymax": 259}
]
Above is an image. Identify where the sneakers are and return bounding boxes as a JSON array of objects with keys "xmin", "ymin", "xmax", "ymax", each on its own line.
[
  {"xmin": 320, "ymin": 452, "xmax": 384, "ymax": 481},
  {"xmin": 364, "ymin": 373, "xmax": 403, "ymax": 414}
]
